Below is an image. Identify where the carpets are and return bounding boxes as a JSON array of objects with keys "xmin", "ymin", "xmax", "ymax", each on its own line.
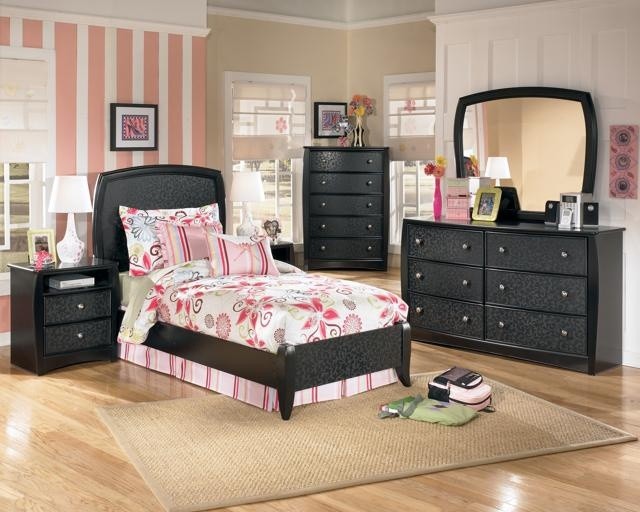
[{"xmin": 93, "ymin": 366, "xmax": 639, "ymax": 512}]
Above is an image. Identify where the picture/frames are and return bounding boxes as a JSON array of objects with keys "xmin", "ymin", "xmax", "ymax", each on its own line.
[
  {"xmin": 315, "ymin": 100, "xmax": 349, "ymax": 139},
  {"xmin": 26, "ymin": 228, "xmax": 58, "ymax": 268},
  {"xmin": 472, "ymin": 188, "xmax": 503, "ymax": 223},
  {"xmin": 109, "ymin": 102, "xmax": 160, "ymax": 152}
]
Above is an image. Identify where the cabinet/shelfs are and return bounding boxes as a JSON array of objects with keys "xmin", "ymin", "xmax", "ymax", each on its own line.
[
  {"xmin": 6, "ymin": 256, "xmax": 122, "ymax": 378},
  {"xmin": 302, "ymin": 144, "xmax": 392, "ymax": 272},
  {"xmin": 398, "ymin": 216, "xmax": 628, "ymax": 376}
]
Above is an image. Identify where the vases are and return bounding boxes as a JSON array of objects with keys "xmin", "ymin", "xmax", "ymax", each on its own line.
[
  {"xmin": 352, "ymin": 116, "xmax": 365, "ymax": 148},
  {"xmin": 433, "ymin": 181, "xmax": 443, "ymax": 220}
]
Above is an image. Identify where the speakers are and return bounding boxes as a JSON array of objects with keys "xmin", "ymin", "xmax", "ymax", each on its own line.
[
  {"xmin": 544, "ymin": 200, "xmax": 559, "ymax": 226},
  {"xmin": 583, "ymin": 201, "xmax": 599, "ymax": 229}
]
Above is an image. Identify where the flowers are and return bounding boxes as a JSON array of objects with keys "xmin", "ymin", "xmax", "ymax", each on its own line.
[
  {"xmin": 423, "ymin": 156, "xmax": 449, "ymax": 179},
  {"xmin": 348, "ymin": 96, "xmax": 375, "ymax": 118}
]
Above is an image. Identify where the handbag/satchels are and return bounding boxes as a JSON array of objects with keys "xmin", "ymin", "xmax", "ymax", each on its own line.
[
  {"xmin": 378, "ymin": 394, "xmax": 480, "ymax": 426},
  {"xmin": 428, "ymin": 366, "xmax": 493, "ymax": 412}
]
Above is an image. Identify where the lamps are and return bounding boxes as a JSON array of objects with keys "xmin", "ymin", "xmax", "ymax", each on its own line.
[
  {"xmin": 47, "ymin": 174, "xmax": 96, "ymax": 265},
  {"xmin": 229, "ymin": 172, "xmax": 266, "ymax": 238},
  {"xmin": 484, "ymin": 157, "xmax": 512, "ymax": 186}
]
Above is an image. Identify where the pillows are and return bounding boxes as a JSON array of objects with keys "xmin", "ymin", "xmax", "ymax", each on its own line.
[{"xmin": 116, "ymin": 204, "xmax": 281, "ymax": 280}]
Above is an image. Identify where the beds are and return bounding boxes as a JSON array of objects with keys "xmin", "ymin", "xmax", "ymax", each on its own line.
[{"xmin": 91, "ymin": 164, "xmax": 412, "ymax": 420}]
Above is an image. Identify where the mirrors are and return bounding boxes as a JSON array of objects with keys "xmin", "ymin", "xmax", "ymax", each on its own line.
[{"xmin": 452, "ymin": 84, "xmax": 597, "ymax": 225}]
